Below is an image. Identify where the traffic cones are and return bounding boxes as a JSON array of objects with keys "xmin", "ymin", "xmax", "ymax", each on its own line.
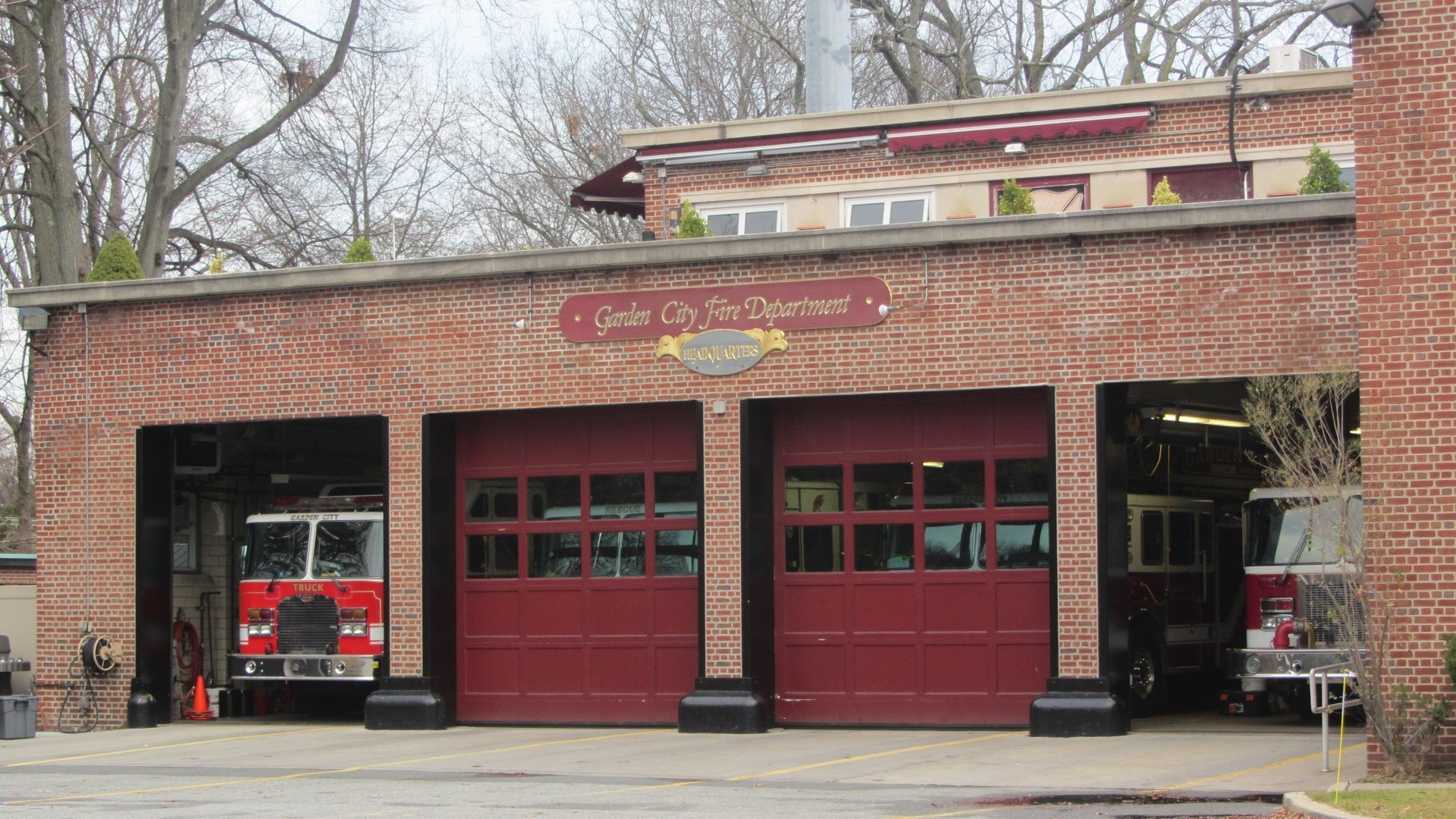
[{"xmin": 188, "ymin": 674, "xmax": 216, "ymax": 721}]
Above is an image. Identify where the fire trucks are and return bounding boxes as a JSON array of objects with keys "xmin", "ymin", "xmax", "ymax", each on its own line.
[
  {"xmin": 881, "ymin": 491, "xmax": 1227, "ymax": 706},
  {"xmin": 538, "ymin": 480, "xmax": 841, "ymax": 579},
  {"xmin": 231, "ymin": 479, "xmax": 547, "ymax": 691},
  {"xmin": 1240, "ymin": 490, "xmax": 1380, "ymax": 694}
]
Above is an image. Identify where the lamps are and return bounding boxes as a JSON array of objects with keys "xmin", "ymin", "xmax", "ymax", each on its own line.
[
  {"xmin": 508, "ymin": 319, "xmax": 530, "ymax": 332},
  {"xmin": 746, "ymin": 164, "xmax": 767, "ymax": 178},
  {"xmin": 1142, "ymin": 406, "xmax": 1251, "ymax": 428},
  {"xmin": 1244, "ymin": 97, "xmax": 1270, "ymax": 112},
  {"xmin": 1004, "ymin": 142, "xmax": 1026, "ymax": 154},
  {"xmin": 912, "ymin": 461, "xmax": 944, "ymax": 469},
  {"xmin": 879, "ymin": 305, "xmax": 903, "ymax": 316},
  {"xmin": 1348, "ymin": 424, "xmax": 1361, "ymax": 435},
  {"xmin": 1321, "ymin": 0, "xmax": 1383, "ymax": 38}
]
